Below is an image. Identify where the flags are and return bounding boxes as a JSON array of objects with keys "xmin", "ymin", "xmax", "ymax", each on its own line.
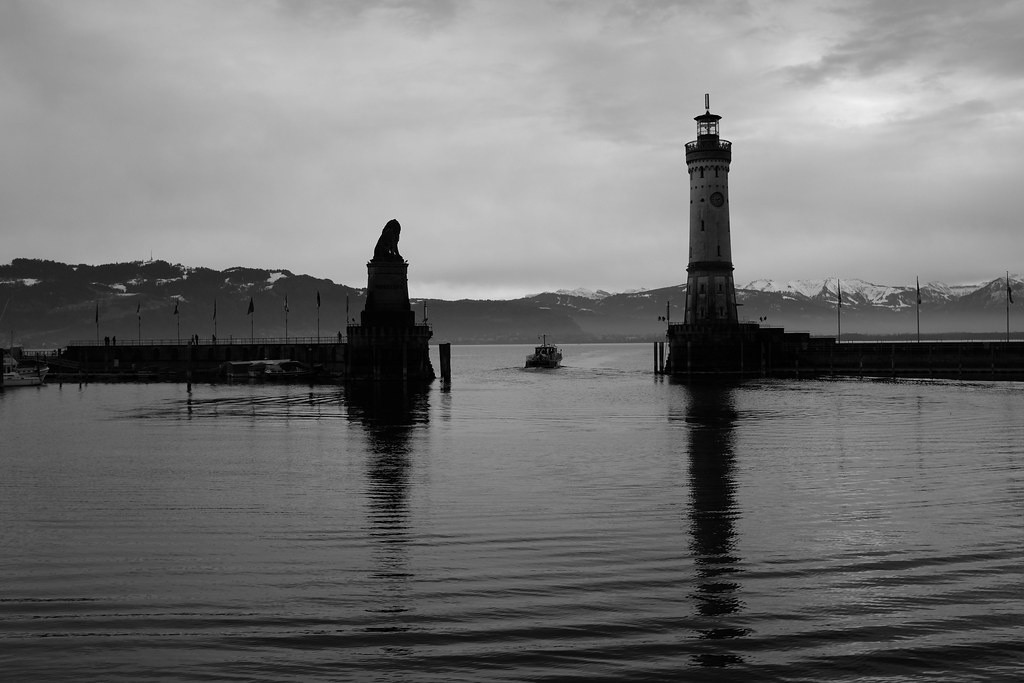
[
  {"xmin": 136, "ymin": 303, "xmax": 141, "ymax": 320},
  {"xmin": 213, "ymin": 299, "xmax": 216, "ymax": 320},
  {"xmin": 95, "ymin": 304, "xmax": 98, "ymax": 323},
  {"xmin": 284, "ymin": 293, "xmax": 289, "ymax": 313},
  {"xmin": 173, "ymin": 299, "xmax": 179, "ymax": 315},
  {"xmin": 316, "ymin": 290, "xmax": 320, "ymax": 308},
  {"xmin": 247, "ymin": 297, "xmax": 254, "ymax": 315}
]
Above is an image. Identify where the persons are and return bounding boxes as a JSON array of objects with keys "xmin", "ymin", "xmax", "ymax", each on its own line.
[
  {"xmin": 191, "ymin": 334, "xmax": 198, "ymax": 345},
  {"xmin": 337, "ymin": 331, "xmax": 342, "ymax": 343},
  {"xmin": 212, "ymin": 334, "xmax": 216, "ymax": 344},
  {"xmin": 104, "ymin": 336, "xmax": 116, "ymax": 345}
]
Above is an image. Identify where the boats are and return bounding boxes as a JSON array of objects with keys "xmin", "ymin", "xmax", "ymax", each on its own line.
[
  {"xmin": 525, "ymin": 332, "xmax": 563, "ymax": 367},
  {"xmin": 0, "ymin": 353, "xmax": 49, "ymax": 386},
  {"xmin": 224, "ymin": 359, "xmax": 324, "ymax": 381}
]
